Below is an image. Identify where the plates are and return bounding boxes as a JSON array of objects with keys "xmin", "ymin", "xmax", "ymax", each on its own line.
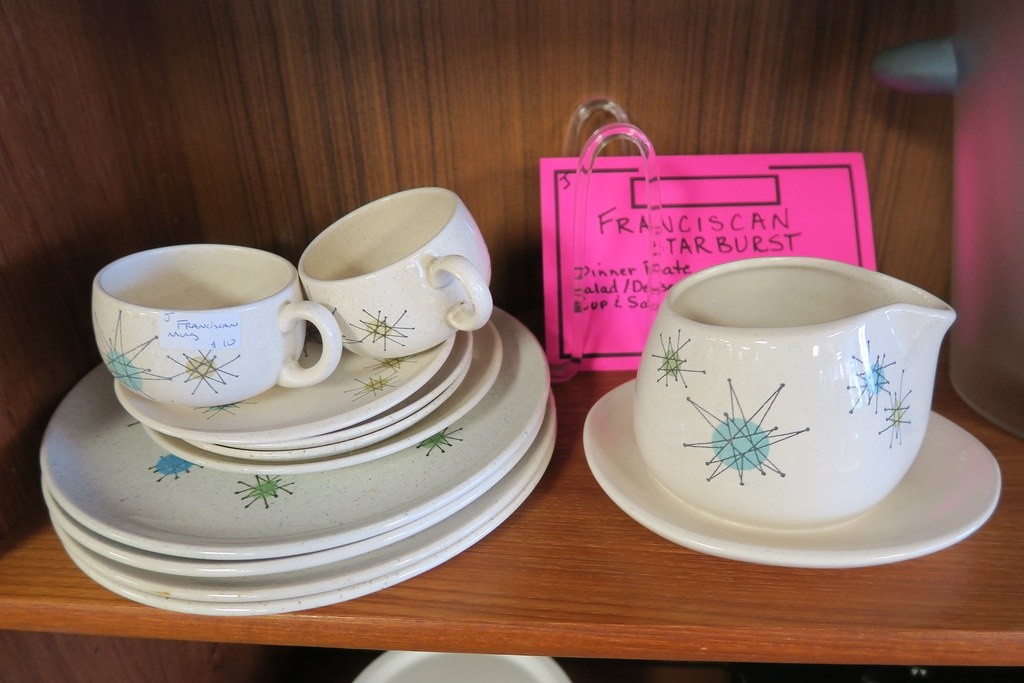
[
  {"xmin": 583, "ymin": 377, "xmax": 1002, "ymax": 571},
  {"xmin": 40, "ymin": 305, "xmax": 559, "ymax": 618}
]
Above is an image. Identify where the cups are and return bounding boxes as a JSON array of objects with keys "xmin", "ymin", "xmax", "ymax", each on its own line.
[
  {"xmin": 297, "ymin": 188, "xmax": 495, "ymax": 359},
  {"xmin": 351, "ymin": 651, "xmax": 570, "ymax": 683},
  {"xmin": 93, "ymin": 245, "xmax": 343, "ymax": 409},
  {"xmin": 947, "ymin": 0, "xmax": 1024, "ymax": 444},
  {"xmin": 632, "ymin": 255, "xmax": 958, "ymax": 526}
]
